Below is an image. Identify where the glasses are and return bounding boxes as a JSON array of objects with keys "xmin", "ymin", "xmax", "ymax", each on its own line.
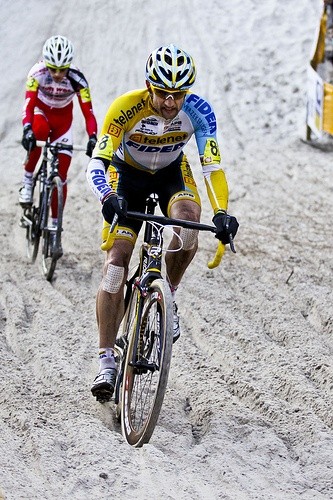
[
  {"xmin": 153, "ymin": 87, "xmax": 188, "ymax": 100},
  {"xmin": 46, "ymin": 66, "xmax": 70, "ymax": 72}
]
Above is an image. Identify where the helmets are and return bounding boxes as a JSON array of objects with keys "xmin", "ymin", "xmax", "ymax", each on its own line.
[
  {"xmin": 145, "ymin": 44, "xmax": 196, "ymax": 90},
  {"xmin": 42, "ymin": 35, "xmax": 73, "ymax": 69}
]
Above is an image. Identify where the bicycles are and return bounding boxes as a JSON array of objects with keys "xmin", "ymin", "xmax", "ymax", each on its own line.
[
  {"xmin": 18, "ymin": 138, "xmax": 87, "ymax": 281},
  {"xmin": 101, "ymin": 193, "xmax": 226, "ymax": 447}
]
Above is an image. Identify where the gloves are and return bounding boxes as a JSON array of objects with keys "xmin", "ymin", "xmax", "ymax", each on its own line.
[
  {"xmin": 21, "ymin": 123, "xmax": 37, "ymax": 152},
  {"xmin": 85, "ymin": 134, "xmax": 97, "ymax": 158},
  {"xmin": 213, "ymin": 214, "xmax": 239, "ymax": 245},
  {"xmin": 101, "ymin": 194, "xmax": 125, "ymax": 223}
]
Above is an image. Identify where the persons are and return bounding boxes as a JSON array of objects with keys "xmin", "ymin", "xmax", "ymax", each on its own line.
[
  {"xmin": 18, "ymin": 35, "xmax": 98, "ymax": 259},
  {"xmin": 85, "ymin": 43, "xmax": 239, "ymax": 400}
]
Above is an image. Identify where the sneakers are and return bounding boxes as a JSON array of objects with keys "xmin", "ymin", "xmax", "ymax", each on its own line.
[
  {"xmin": 19, "ymin": 174, "xmax": 33, "ymax": 208},
  {"xmin": 159, "ymin": 301, "xmax": 181, "ymax": 344},
  {"xmin": 90, "ymin": 362, "xmax": 117, "ymax": 401},
  {"xmin": 52, "ymin": 219, "xmax": 63, "ymax": 259}
]
